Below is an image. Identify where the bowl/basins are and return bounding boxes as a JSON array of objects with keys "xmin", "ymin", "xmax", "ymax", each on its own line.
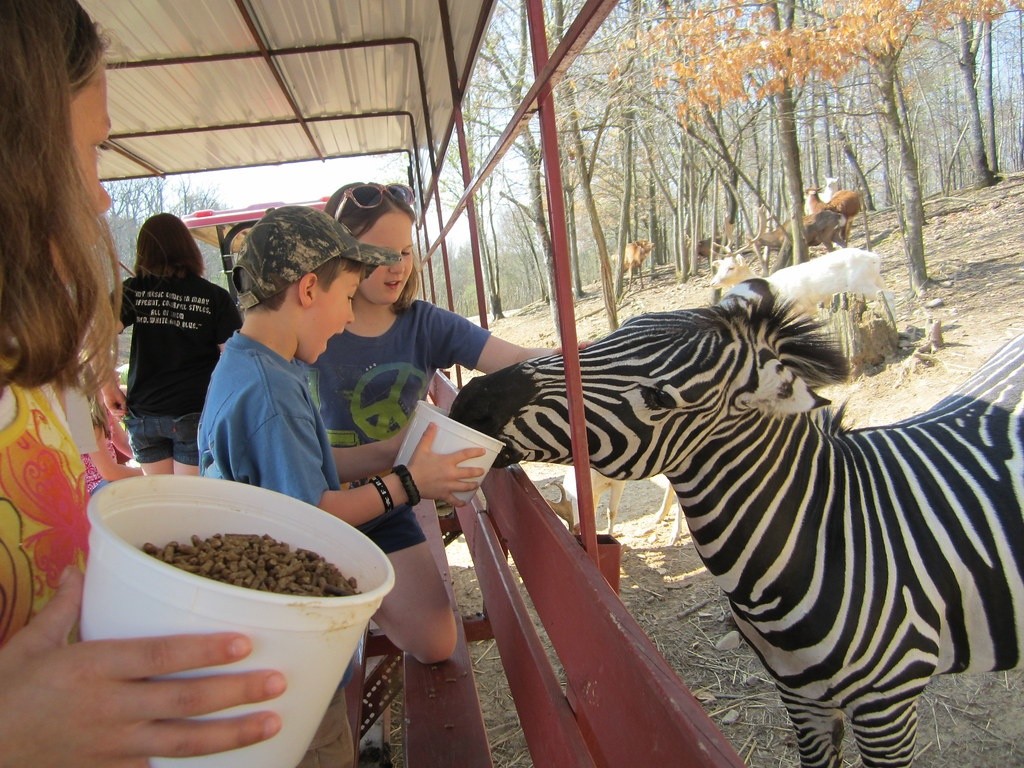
[
  {"xmin": 392, "ymin": 399, "xmax": 506, "ymax": 505},
  {"xmin": 78, "ymin": 475, "xmax": 395, "ymax": 768}
]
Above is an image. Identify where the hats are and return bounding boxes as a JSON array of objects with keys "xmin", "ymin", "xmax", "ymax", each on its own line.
[{"xmin": 231, "ymin": 205, "xmax": 401, "ymax": 309}]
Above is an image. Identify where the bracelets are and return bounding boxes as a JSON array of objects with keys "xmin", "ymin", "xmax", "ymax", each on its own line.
[
  {"xmin": 391, "ymin": 464, "xmax": 421, "ymax": 506},
  {"xmin": 369, "ymin": 475, "xmax": 393, "ymax": 514}
]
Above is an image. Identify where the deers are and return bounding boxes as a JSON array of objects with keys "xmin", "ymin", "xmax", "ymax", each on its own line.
[
  {"xmin": 685, "ymin": 231, "xmax": 736, "ymax": 266},
  {"xmin": 707, "ymin": 205, "xmax": 899, "ymax": 341},
  {"xmin": 751, "ymin": 205, "xmax": 848, "ymax": 261}
]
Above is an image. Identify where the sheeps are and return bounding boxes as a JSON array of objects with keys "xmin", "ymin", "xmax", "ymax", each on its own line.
[
  {"xmin": 609, "ymin": 238, "xmax": 656, "ymax": 292},
  {"xmin": 802, "ymin": 174, "xmax": 860, "ymax": 242}
]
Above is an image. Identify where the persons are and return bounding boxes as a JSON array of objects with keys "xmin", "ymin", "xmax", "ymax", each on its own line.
[
  {"xmin": 0, "ymin": 0, "xmax": 287, "ymax": 768},
  {"xmin": 112, "ymin": 212, "xmax": 243, "ymax": 477},
  {"xmin": 311, "ymin": 183, "xmax": 591, "ymax": 664},
  {"xmin": 197, "ymin": 204, "xmax": 485, "ymax": 768}
]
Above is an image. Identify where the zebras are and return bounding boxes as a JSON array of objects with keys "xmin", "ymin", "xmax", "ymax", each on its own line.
[{"xmin": 448, "ymin": 293, "xmax": 1023, "ymax": 766}]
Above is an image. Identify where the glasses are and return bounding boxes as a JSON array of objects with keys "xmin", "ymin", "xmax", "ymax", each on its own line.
[{"xmin": 335, "ymin": 183, "xmax": 415, "ymax": 224}]
